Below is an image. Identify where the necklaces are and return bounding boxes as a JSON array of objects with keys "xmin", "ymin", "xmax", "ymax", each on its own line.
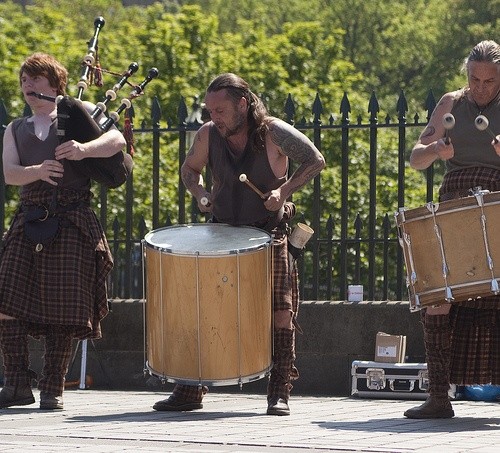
[{"xmin": 467, "ymin": 93, "xmax": 500, "ymax": 115}]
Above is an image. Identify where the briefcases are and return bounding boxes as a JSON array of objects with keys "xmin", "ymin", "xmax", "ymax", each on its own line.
[{"xmin": 352, "ymin": 359, "xmax": 430, "ymax": 399}]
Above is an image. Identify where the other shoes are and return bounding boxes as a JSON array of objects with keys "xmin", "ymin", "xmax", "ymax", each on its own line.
[
  {"xmin": 404, "ymin": 391, "xmax": 455, "ymax": 418},
  {"xmin": 266, "ymin": 394, "xmax": 290, "ymax": 415},
  {"xmin": 154, "ymin": 395, "xmax": 204, "ymax": 412},
  {"xmin": 39, "ymin": 390, "xmax": 62, "ymax": 409},
  {"xmin": 0, "ymin": 384, "xmax": 35, "ymax": 408}
]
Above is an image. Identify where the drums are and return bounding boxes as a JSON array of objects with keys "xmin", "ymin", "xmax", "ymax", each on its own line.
[
  {"xmin": 145, "ymin": 224, "xmax": 272, "ymax": 388},
  {"xmin": 395, "ymin": 187, "xmax": 500, "ymax": 312}
]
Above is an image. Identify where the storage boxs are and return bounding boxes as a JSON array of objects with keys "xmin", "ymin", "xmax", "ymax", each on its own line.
[
  {"xmin": 352, "ymin": 360, "xmax": 464, "ymax": 401},
  {"xmin": 374, "ymin": 334, "xmax": 403, "ymax": 364},
  {"xmin": 349, "ymin": 285, "xmax": 363, "ymax": 302},
  {"xmin": 377, "ymin": 331, "xmax": 407, "ymax": 364}
]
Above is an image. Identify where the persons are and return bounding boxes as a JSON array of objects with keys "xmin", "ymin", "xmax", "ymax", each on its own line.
[
  {"xmin": 0, "ymin": 51, "xmax": 127, "ymax": 409},
  {"xmin": 152, "ymin": 73, "xmax": 326, "ymax": 415},
  {"xmin": 403, "ymin": 40, "xmax": 500, "ymax": 419}
]
